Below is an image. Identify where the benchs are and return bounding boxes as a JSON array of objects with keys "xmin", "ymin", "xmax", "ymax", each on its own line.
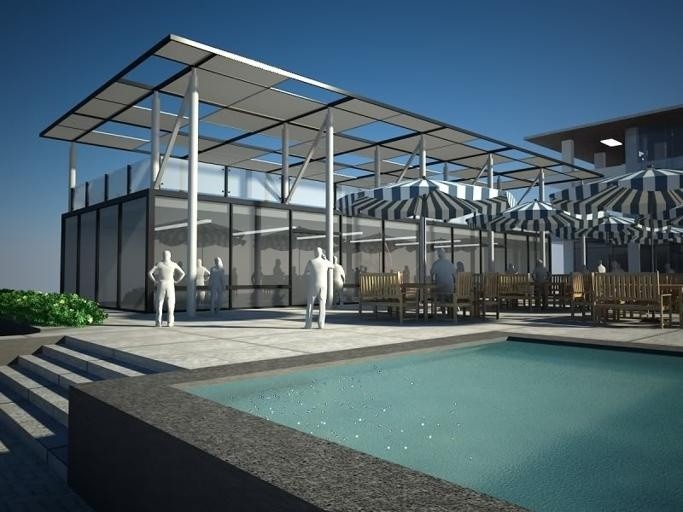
[{"xmin": 353, "ymin": 263, "xmax": 682, "ymax": 331}]
